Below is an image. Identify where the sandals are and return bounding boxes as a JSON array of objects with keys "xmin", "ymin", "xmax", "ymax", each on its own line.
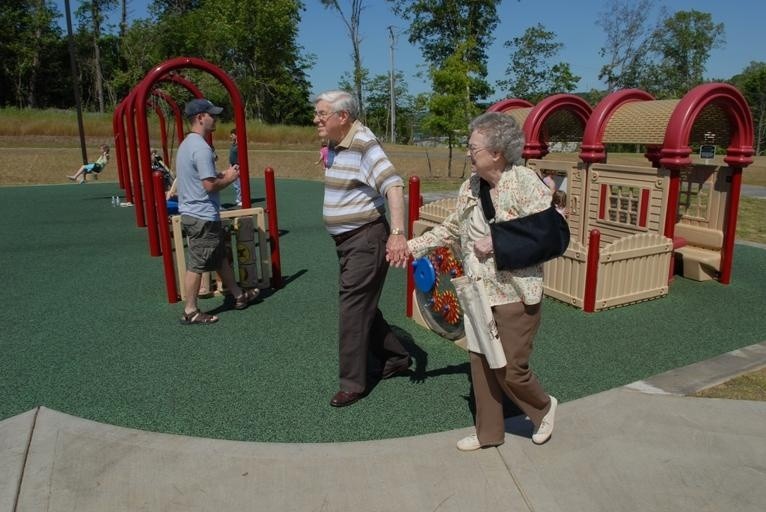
[
  {"xmin": 232, "ymin": 288, "xmax": 260, "ymax": 310},
  {"xmin": 180, "ymin": 308, "xmax": 218, "ymax": 325}
]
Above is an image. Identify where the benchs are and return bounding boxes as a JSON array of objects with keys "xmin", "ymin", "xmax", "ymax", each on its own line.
[{"xmin": 673, "ymin": 223, "xmax": 724, "ymax": 281}]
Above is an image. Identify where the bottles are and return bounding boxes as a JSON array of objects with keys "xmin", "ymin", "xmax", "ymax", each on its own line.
[{"xmin": 112, "ymin": 195, "xmax": 120, "ymax": 206}]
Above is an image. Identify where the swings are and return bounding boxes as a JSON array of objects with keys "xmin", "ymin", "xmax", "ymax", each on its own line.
[
  {"xmin": 161, "ymin": 105, "xmax": 177, "ymax": 191},
  {"xmin": 90, "ymin": 123, "xmax": 120, "ymax": 180}
]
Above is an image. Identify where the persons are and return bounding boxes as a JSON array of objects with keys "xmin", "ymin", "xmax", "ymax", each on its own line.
[
  {"xmin": 314, "ymin": 142, "xmax": 329, "ymax": 169},
  {"xmin": 550, "ymin": 189, "xmax": 567, "ymax": 220},
  {"xmin": 150, "ymin": 150, "xmax": 157, "ymax": 163},
  {"xmin": 229, "ymin": 128, "xmax": 243, "ymax": 207},
  {"xmin": 66, "ymin": 143, "xmax": 110, "ymax": 184},
  {"xmin": 176, "ymin": 98, "xmax": 260, "ymax": 325},
  {"xmin": 385, "ymin": 111, "xmax": 571, "ymax": 451},
  {"xmin": 543, "ymin": 173, "xmax": 556, "ymax": 195},
  {"xmin": 310, "ymin": 89, "xmax": 414, "ymax": 408},
  {"xmin": 151, "ymin": 155, "xmax": 174, "ymax": 191}
]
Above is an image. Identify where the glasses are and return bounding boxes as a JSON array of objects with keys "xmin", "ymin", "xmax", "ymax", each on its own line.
[
  {"xmin": 312, "ymin": 111, "xmax": 338, "ymax": 122},
  {"xmin": 464, "ymin": 143, "xmax": 492, "ymax": 154}
]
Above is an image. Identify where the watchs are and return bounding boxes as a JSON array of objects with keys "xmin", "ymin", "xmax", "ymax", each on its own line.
[{"xmin": 390, "ymin": 228, "xmax": 405, "ymax": 235}]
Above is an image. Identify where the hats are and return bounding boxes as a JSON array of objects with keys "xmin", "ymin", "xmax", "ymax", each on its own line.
[{"xmin": 183, "ymin": 98, "xmax": 224, "ymax": 117}]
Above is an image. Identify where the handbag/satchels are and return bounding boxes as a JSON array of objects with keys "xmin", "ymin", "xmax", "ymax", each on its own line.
[{"xmin": 450, "ymin": 242, "xmax": 507, "ymax": 369}]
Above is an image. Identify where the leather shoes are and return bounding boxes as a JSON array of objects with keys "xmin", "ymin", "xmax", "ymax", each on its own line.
[
  {"xmin": 330, "ymin": 389, "xmax": 361, "ymax": 407},
  {"xmin": 374, "ymin": 367, "xmax": 394, "ymax": 378}
]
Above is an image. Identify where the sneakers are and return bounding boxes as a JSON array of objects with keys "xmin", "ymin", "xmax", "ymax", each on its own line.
[
  {"xmin": 531, "ymin": 394, "xmax": 558, "ymax": 444},
  {"xmin": 456, "ymin": 434, "xmax": 480, "ymax": 452}
]
